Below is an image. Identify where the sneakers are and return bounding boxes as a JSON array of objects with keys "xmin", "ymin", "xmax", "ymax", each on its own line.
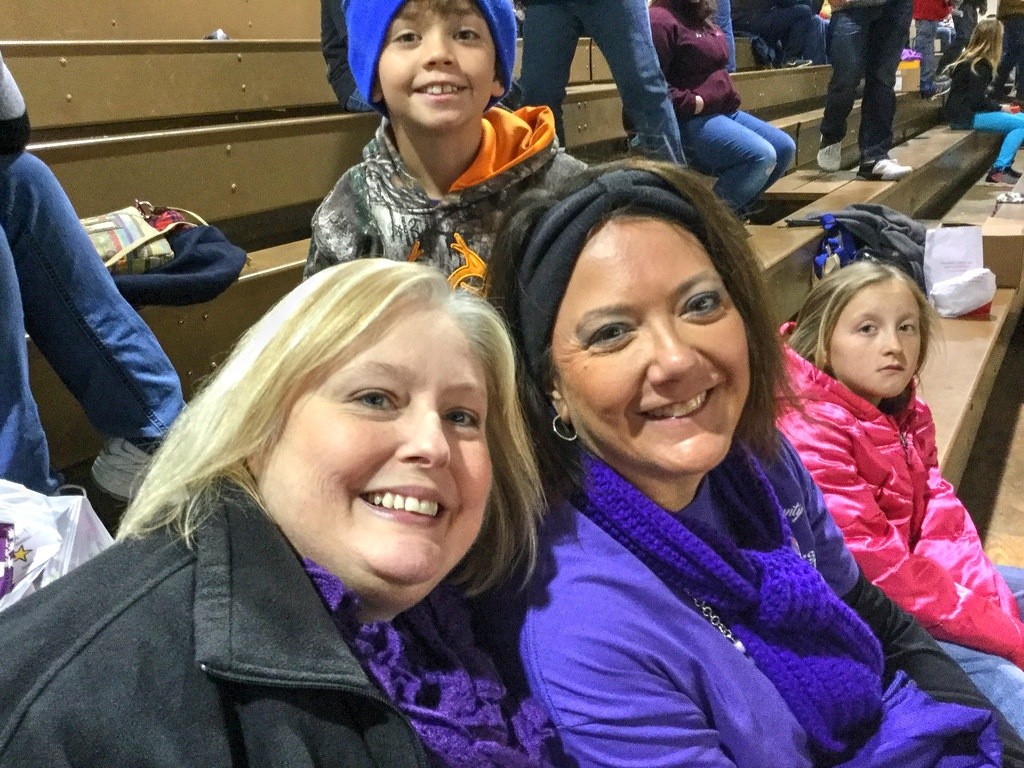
[
  {"xmin": 856, "ymin": 157, "xmax": 912, "ymax": 180},
  {"xmin": 815, "ymin": 134, "xmax": 843, "ymax": 173}
]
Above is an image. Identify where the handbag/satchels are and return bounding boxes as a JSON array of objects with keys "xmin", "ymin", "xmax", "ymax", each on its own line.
[{"xmin": 77, "ymin": 202, "xmax": 210, "ymax": 275}]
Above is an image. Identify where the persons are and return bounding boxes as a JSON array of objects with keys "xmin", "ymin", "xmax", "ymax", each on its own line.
[
  {"xmin": 713, "ymin": 0, "xmax": 828, "ymax": 73},
  {"xmin": 774, "ymin": 261, "xmax": 1024, "ymax": 742},
  {"xmin": 622, "ymin": 0, "xmax": 796, "ymax": 218},
  {"xmin": 0, "ymin": 256, "xmax": 567, "ymax": 768},
  {"xmin": 482, "ymin": 158, "xmax": 1024, "ymax": 768},
  {"xmin": 0, "ymin": 52, "xmax": 195, "ymax": 504},
  {"xmin": 300, "ymin": 0, "xmax": 591, "ymax": 299},
  {"xmin": 817, "ymin": 0, "xmax": 913, "ymax": 181},
  {"xmin": 913, "ymin": 0, "xmax": 1024, "ymax": 187},
  {"xmin": 320, "ymin": 0, "xmax": 688, "ymax": 171}
]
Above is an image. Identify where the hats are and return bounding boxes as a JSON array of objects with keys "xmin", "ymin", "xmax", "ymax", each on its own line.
[{"xmin": 344, "ymin": 0, "xmax": 517, "ymax": 114}]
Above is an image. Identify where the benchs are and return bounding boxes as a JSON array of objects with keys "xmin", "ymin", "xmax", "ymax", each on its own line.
[{"xmin": 0, "ymin": 0, "xmax": 1024, "ymax": 498}]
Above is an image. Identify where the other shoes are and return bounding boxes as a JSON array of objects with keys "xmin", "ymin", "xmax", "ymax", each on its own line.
[
  {"xmin": 783, "ymin": 58, "xmax": 813, "ymax": 68},
  {"xmin": 985, "ymin": 166, "xmax": 1022, "ymax": 187},
  {"xmin": 928, "ymin": 73, "xmax": 952, "ymax": 99},
  {"xmin": 90, "ymin": 436, "xmax": 159, "ymax": 503}
]
